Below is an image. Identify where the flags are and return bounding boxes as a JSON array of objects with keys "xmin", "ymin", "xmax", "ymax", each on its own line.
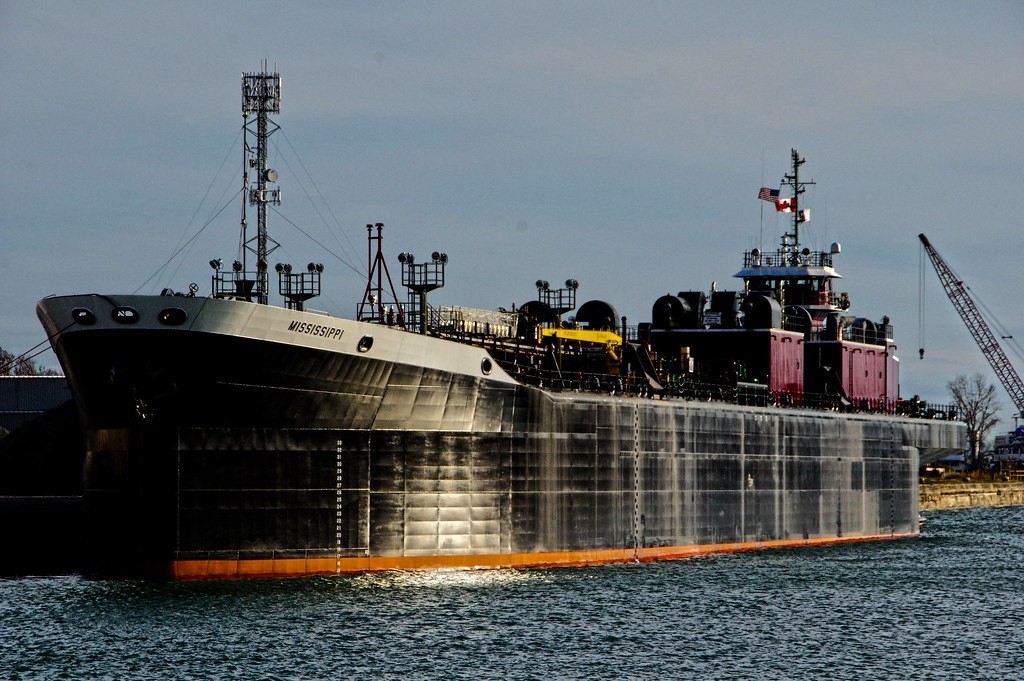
[
  {"xmin": 775, "ymin": 197, "xmax": 798, "ymax": 213},
  {"xmin": 797, "ymin": 209, "xmax": 810, "ymax": 224},
  {"xmin": 758, "ymin": 187, "xmax": 780, "ymax": 203}
]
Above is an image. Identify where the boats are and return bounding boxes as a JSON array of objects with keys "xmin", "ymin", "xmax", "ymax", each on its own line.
[{"xmin": 35, "ymin": 59, "xmax": 922, "ymax": 579}]
